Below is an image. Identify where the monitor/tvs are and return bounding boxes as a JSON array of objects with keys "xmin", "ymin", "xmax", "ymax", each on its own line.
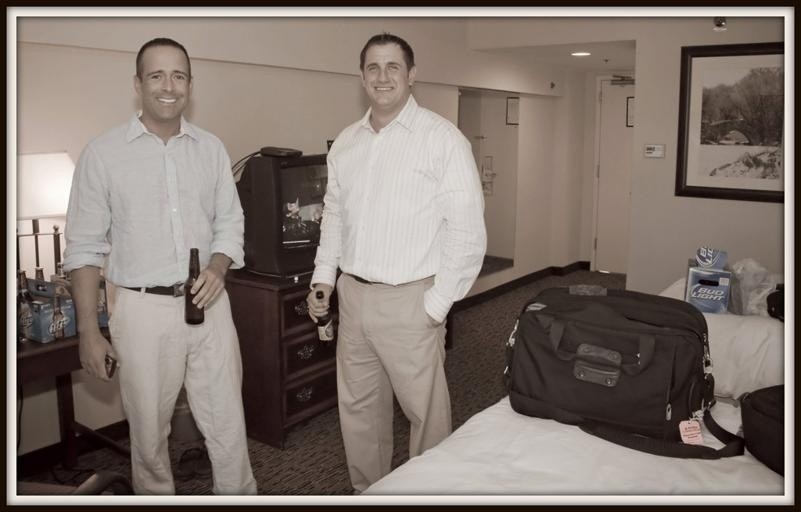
[{"xmin": 234, "ymin": 153, "xmax": 328, "ymax": 280}]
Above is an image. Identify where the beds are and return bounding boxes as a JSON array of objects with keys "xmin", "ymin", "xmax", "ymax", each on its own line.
[{"xmin": 360, "ymin": 275, "xmax": 788, "ymax": 494}]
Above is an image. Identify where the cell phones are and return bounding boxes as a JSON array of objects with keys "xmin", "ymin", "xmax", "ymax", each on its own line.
[{"xmin": 106, "ymin": 355, "xmax": 116, "ymax": 378}]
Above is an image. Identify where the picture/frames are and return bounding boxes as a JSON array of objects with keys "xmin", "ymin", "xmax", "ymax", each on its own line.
[{"xmin": 675, "ymin": 40, "xmax": 786, "ymax": 202}]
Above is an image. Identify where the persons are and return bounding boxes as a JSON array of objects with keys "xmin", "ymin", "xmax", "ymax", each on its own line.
[
  {"xmin": 306, "ymin": 32, "xmax": 488, "ymax": 495},
  {"xmin": 63, "ymin": 39, "xmax": 258, "ymax": 495}
]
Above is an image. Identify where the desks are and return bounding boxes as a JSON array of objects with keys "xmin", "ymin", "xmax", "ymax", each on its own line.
[{"xmin": 18, "ymin": 328, "xmax": 132, "ymax": 466}]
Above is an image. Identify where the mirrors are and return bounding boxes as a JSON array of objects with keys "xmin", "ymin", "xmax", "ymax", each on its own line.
[{"xmin": 459, "ymin": 83, "xmax": 523, "ymax": 278}]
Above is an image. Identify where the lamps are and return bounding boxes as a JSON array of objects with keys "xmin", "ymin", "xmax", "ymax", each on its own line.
[{"xmin": 17, "ymin": 151, "xmax": 77, "ymax": 283}]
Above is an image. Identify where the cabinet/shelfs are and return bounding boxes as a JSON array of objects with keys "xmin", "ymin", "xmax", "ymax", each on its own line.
[{"xmin": 223, "ymin": 270, "xmax": 339, "ymax": 448}]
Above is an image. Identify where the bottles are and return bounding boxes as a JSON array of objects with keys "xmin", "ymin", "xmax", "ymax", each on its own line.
[
  {"xmin": 316, "ymin": 290, "xmax": 335, "ymax": 352},
  {"xmin": 184, "ymin": 248, "xmax": 205, "ymax": 325},
  {"xmin": 35, "ymin": 266, "xmax": 46, "ymax": 292},
  {"xmin": 52, "ymin": 294, "xmax": 65, "ymax": 340},
  {"xmin": 16, "ymin": 269, "xmax": 35, "ymax": 344}
]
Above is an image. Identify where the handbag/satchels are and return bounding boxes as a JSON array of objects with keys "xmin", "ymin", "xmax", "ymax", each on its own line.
[{"xmin": 505, "ymin": 281, "xmax": 720, "ymax": 441}]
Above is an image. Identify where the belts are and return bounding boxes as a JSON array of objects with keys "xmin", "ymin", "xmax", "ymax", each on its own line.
[
  {"xmin": 345, "ymin": 274, "xmax": 384, "ymax": 286},
  {"xmin": 109, "ymin": 278, "xmax": 185, "ymax": 298}
]
